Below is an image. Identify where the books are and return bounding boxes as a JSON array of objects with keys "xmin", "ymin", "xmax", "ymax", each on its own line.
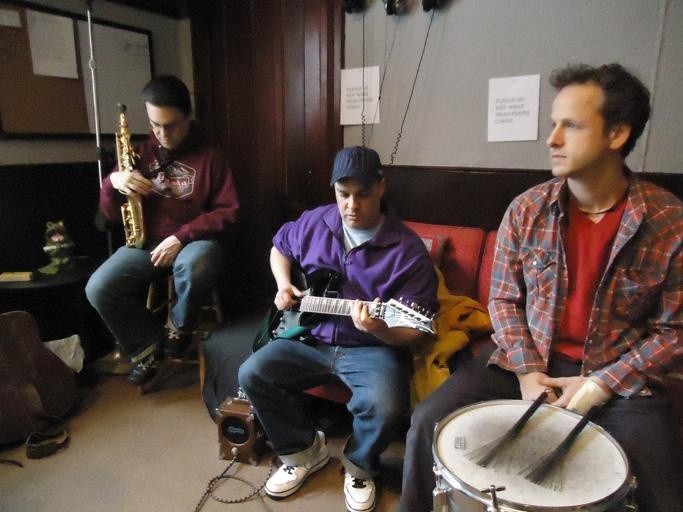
[{"xmin": 0, "ymin": 269, "xmax": 34, "ymax": 284}]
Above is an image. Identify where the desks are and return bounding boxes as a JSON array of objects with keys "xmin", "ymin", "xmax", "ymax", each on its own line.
[{"xmin": 0, "ymin": 260, "xmax": 104, "ymax": 354}]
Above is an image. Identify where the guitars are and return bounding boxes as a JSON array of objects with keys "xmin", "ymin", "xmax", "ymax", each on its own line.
[{"xmin": 268, "ymin": 266, "xmax": 439, "ymax": 339}]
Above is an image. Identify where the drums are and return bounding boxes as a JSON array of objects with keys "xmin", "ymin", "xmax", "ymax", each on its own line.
[{"xmin": 431, "ymin": 399, "xmax": 638, "ymax": 512}]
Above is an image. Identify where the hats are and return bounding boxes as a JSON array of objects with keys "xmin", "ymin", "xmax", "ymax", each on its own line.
[{"xmin": 329, "ymin": 145, "xmax": 381, "ymax": 187}]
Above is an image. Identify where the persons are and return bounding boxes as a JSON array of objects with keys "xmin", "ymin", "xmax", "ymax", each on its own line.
[
  {"xmin": 238, "ymin": 146, "xmax": 442, "ymax": 510},
  {"xmin": 393, "ymin": 59, "xmax": 683, "ymax": 511},
  {"xmin": 82, "ymin": 73, "xmax": 242, "ymax": 386}
]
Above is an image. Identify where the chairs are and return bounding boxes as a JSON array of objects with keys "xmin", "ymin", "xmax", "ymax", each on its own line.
[{"xmin": 291, "ymin": 216, "xmax": 497, "ymax": 418}]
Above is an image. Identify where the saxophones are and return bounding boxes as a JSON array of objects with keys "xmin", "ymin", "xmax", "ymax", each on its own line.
[{"xmin": 114, "ymin": 100, "xmax": 148, "ymax": 249}]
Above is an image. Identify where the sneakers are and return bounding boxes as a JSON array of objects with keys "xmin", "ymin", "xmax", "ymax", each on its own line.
[
  {"xmin": 264, "ymin": 430, "xmax": 330, "ymax": 498},
  {"xmin": 126, "ymin": 347, "xmax": 164, "ymax": 386},
  {"xmin": 162, "ymin": 322, "xmax": 192, "ymax": 357},
  {"xmin": 343, "ymin": 468, "xmax": 378, "ymax": 512}
]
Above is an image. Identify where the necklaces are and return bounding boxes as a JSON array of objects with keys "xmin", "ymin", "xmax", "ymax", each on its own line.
[{"xmin": 574, "ymin": 192, "xmax": 617, "ymax": 217}]
